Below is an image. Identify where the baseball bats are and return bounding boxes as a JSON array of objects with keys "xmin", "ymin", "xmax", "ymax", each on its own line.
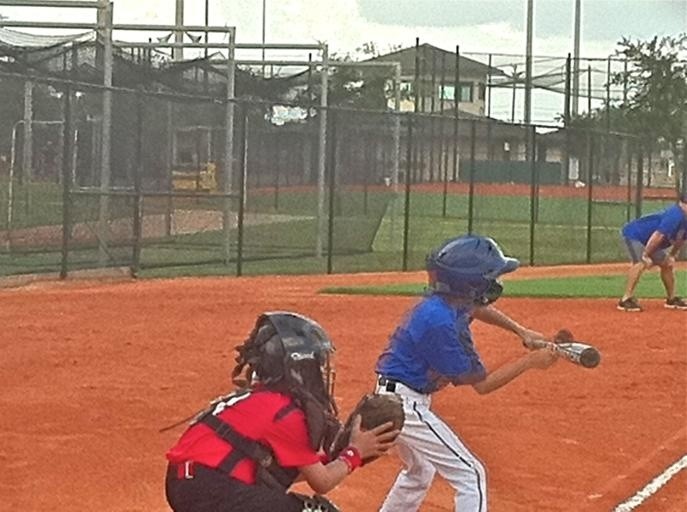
[{"xmin": 533, "ymin": 339, "xmax": 600, "ymax": 368}]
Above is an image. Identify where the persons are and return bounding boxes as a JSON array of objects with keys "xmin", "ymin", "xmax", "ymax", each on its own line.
[
  {"xmin": 165, "ymin": 312, "xmax": 402, "ymax": 511},
  {"xmin": 616, "ymin": 195, "xmax": 686, "ymax": 312},
  {"xmin": 375, "ymin": 235, "xmax": 558, "ymax": 511}
]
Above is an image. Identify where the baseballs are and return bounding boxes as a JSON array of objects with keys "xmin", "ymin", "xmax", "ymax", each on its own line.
[{"xmin": 554, "ymin": 330, "xmax": 574, "ymax": 342}]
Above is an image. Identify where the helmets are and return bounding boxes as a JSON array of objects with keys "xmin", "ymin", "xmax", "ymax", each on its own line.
[
  {"xmin": 249, "ymin": 312, "xmax": 333, "ymax": 390},
  {"xmin": 430, "ymin": 237, "xmax": 520, "ymax": 298}
]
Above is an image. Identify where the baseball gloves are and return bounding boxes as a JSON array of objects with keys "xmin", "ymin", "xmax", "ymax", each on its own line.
[{"xmin": 322, "ymin": 393, "xmax": 404, "ymax": 466}]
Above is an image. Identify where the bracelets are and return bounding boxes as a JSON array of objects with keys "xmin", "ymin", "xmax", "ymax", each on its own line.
[{"xmin": 340, "ymin": 447, "xmax": 362, "ymax": 474}]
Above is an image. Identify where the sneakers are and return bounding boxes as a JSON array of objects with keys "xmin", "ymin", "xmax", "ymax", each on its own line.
[
  {"xmin": 617, "ymin": 297, "xmax": 640, "ymax": 312},
  {"xmin": 664, "ymin": 297, "xmax": 687, "ymax": 310}
]
